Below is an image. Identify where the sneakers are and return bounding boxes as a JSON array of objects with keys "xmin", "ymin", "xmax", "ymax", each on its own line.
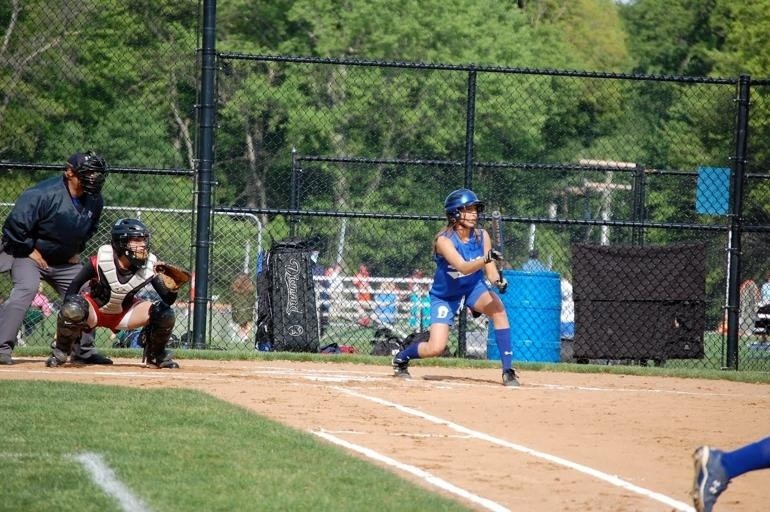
[
  {"xmin": 501, "ymin": 367, "xmax": 521, "ymax": 386},
  {"xmin": 45, "ymin": 351, "xmax": 67, "ymax": 368},
  {"xmin": 390, "ymin": 351, "xmax": 413, "ymax": 380},
  {"xmin": 146, "ymin": 354, "xmax": 180, "ymax": 368},
  {"xmin": 70, "ymin": 351, "xmax": 114, "ymax": 365},
  {"xmin": 0, "ymin": 344, "xmax": 16, "ymax": 367},
  {"xmin": 691, "ymin": 445, "xmax": 732, "ymax": 512}
]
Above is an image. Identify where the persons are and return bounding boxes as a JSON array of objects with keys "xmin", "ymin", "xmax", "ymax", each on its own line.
[
  {"xmin": 18, "ymin": 283, "xmax": 48, "ymax": 345},
  {"xmin": 314, "ymin": 263, "xmax": 436, "ymax": 338},
  {"xmin": 736, "ymin": 269, "xmax": 770, "ymax": 344},
  {"xmin": 48, "ymin": 218, "xmax": 190, "ymax": 368},
  {"xmin": 391, "ymin": 186, "xmax": 525, "ymax": 389},
  {"xmin": 0, "ymin": 151, "xmax": 113, "ymax": 366},
  {"xmin": 685, "ymin": 434, "xmax": 770, "ymax": 512}
]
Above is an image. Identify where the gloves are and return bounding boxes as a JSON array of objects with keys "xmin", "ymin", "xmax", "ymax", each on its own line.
[
  {"xmin": 482, "ymin": 246, "xmax": 504, "ymax": 265},
  {"xmin": 493, "ymin": 277, "xmax": 508, "ymax": 294}
]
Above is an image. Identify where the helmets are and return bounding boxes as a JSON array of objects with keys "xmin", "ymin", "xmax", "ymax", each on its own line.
[
  {"xmin": 443, "ymin": 187, "xmax": 487, "ymax": 219},
  {"xmin": 109, "ymin": 214, "xmax": 150, "ymax": 258},
  {"xmin": 65, "ymin": 149, "xmax": 109, "ymax": 200}
]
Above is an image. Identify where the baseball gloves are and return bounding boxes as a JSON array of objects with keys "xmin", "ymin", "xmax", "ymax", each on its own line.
[{"xmin": 153, "ymin": 264, "xmax": 191, "ymax": 305}]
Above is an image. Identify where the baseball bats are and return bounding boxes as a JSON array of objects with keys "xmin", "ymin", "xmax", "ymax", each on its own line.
[{"xmin": 492, "ymin": 210, "xmax": 505, "ymax": 293}]
